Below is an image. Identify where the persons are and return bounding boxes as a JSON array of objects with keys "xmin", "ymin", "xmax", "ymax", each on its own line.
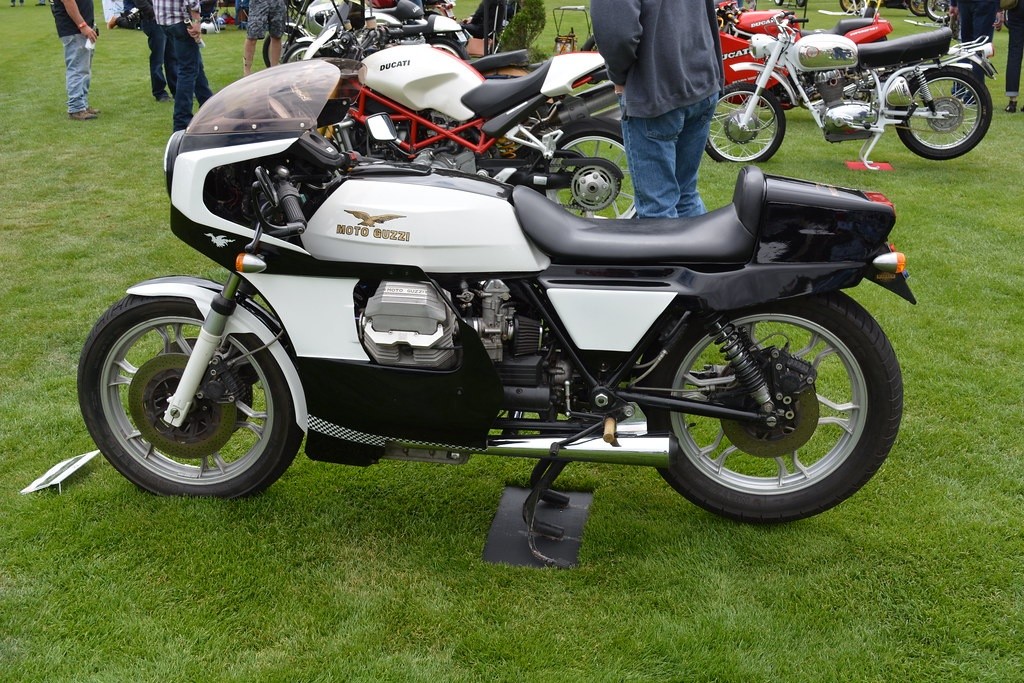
[
  {"xmin": 35, "ymin": 0, "xmax": 54, "ymax": 6},
  {"xmin": 243, "ymin": 0, "xmax": 288, "ymax": 76},
  {"xmin": 588, "ymin": 0, "xmax": 726, "ymax": 219},
  {"xmin": 1005, "ymin": 0, "xmax": 1024, "ymax": 113},
  {"xmin": 460, "ymin": 0, "xmax": 522, "ymax": 39},
  {"xmin": 133, "ymin": 0, "xmax": 196, "ymax": 103},
  {"xmin": 948, "ymin": 0, "xmax": 1004, "ymax": 105},
  {"xmin": 10, "ymin": 0, "xmax": 24, "ymax": 7},
  {"xmin": 151, "ymin": 0, "xmax": 213, "ymax": 134},
  {"xmin": 106, "ymin": 0, "xmax": 144, "ymax": 31},
  {"xmin": 53, "ymin": 0, "xmax": 101, "ymax": 119}
]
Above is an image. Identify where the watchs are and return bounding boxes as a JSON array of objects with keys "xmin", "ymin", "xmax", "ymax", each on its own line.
[
  {"xmin": 77, "ymin": 21, "xmax": 88, "ymax": 28},
  {"xmin": 191, "ymin": 17, "xmax": 202, "ymax": 23}
]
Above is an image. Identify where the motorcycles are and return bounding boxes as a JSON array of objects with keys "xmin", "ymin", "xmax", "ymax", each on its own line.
[
  {"xmin": 73, "ymin": 55, "xmax": 918, "ymax": 574},
  {"xmin": 712, "ymin": 0, "xmax": 958, "ymax": 27},
  {"xmin": 701, "ymin": 11, "xmax": 1000, "ymax": 174},
  {"xmin": 712, "ymin": 0, "xmax": 895, "ymax": 112},
  {"xmin": 292, "ymin": 0, "xmax": 641, "ymax": 221},
  {"xmin": 260, "ymin": 1, "xmax": 547, "ymax": 158}
]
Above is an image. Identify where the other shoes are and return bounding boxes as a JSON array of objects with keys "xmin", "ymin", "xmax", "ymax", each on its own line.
[
  {"xmin": 86, "ymin": 106, "xmax": 100, "ymax": 114},
  {"xmin": 1007, "ymin": 101, "xmax": 1018, "ymax": 112},
  {"xmin": 68, "ymin": 111, "xmax": 98, "ymax": 120},
  {"xmin": 109, "ymin": 16, "xmax": 118, "ymax": 29},
  {"xmin": 158, "ymin": 97, "xmax": 174, "ymax": 102}
]
